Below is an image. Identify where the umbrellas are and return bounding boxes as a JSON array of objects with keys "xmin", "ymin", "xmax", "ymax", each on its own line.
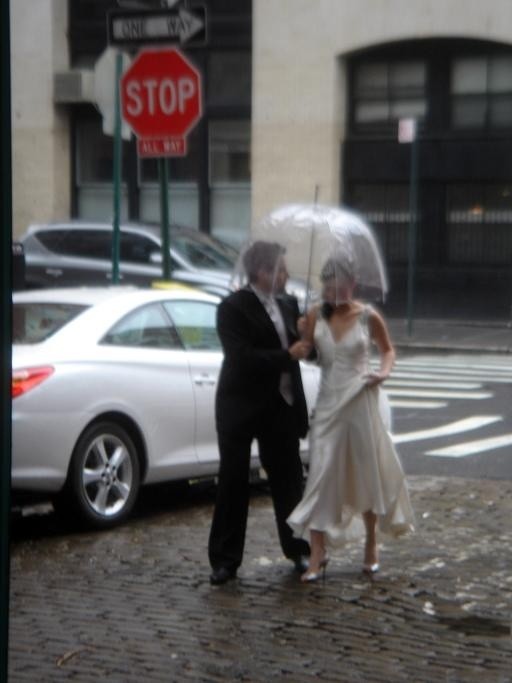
[{"xmin": 225, "ymin": 180, "xmax": 393, "ymax": 347}]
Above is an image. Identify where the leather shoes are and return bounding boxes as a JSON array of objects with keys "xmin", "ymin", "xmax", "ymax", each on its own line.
[
  {"xmin": 296, "ymin": 556, "xmax": 310, "ymax": 571},
  {"xmin": 208, "ymin": 567, "xmax": 235, "ymax": 585}
]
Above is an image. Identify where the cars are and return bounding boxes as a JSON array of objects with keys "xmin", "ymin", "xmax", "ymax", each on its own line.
[
  {"xmin": 11, "ymin": 284, "xmax": 389, "ymax": 533},
  {"xmin": 19, "ymin": 222, "xmax": 324, "ymax": 314}
]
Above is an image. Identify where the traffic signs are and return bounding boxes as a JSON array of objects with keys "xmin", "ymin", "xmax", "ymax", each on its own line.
[{"xmin": 103, "ymin": 7, "xmax": 208, "ymax": 46}]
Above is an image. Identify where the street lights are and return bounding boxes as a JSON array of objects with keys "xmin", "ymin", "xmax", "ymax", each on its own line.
[{"xmin": 397, "ymin": 118, "xmax": 421, "ymax": 335}]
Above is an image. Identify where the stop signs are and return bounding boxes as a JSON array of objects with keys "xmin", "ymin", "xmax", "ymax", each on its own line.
[{"xmin": 118, "ymin": 46, "xmax": 202, "ymax": 159}]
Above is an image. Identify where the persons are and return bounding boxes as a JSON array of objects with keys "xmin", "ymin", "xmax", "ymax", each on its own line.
[
  {"xmin": 295, "ymin": 250, "xmax": 396, "ymax": 584},
  {"xmin": 207, "ymin": 242, "xmax": 316, "ymax": 585}
]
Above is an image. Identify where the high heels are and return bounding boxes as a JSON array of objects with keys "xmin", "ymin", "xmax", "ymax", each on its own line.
[
  {"xmin": 301, "ymin": 556, "xmax": 331, "ymax": 584},
  {"xmin": 362, "ymin": 547, "xmax": 380, "ymax": 573}
]
